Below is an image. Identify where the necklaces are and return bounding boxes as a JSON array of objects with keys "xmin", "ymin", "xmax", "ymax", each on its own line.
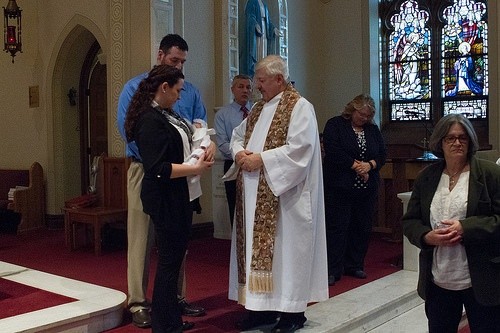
[{"xmin": 446, "ymin": 166, "xmax": 463, "ymax": 186}]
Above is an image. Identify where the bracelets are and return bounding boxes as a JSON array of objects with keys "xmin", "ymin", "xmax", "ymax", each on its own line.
[{"xmin": 368, "ymin": 161, "xmax": 373, "ymax": 169}]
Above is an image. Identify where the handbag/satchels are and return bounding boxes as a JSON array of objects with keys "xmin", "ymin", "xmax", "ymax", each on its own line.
[{"xmin": 64, "ymin": 192, "xmax": 103, "ymax": 209}]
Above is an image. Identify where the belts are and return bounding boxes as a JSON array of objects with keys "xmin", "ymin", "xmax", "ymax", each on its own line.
[{"xmin": 131, "ymin": 156, "xmax": 144, "ymax": 163}]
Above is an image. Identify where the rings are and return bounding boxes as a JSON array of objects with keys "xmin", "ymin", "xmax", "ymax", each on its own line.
[{"xmin": 211, "ymin": 158, "xmax": 214, "ymax": 160}]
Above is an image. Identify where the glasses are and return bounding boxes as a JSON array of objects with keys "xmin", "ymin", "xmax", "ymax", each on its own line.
[
  {"xmin": 358, "ymin": 111, "xmax": 374, "ymax": 121},
  {"xmin": 444, "ymin": 134, "xmax": 469, "ymax": 144}
]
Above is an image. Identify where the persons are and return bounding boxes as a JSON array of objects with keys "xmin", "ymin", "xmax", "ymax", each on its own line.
[
  {"xmin": 401, "ymin": 114, "xmax": 500, "ymax": 333},
  {"xmin": 117, "ymin": 34, "xmax": 208, "ymax": 326},
  {"xmin": 243, "ymin": 0, "xmax": 284, "ymax": 78},
  {"xmin": 323, "ymin": 93, "xmax": 386, "ymax": 285},
  {"xmin": 123, "ymin": 65, "xmax": 216, "ymax": 333},
  {"xmin": 182, "ymin": 118, "xmax": 216, "ymax": 182},
  {"xmin": 213, "ymin": 75, "xmax": 256, "ymax": 229},
  {"xmin": 220, "ymin": 55, "xmax": 330, "ymax": 333}
]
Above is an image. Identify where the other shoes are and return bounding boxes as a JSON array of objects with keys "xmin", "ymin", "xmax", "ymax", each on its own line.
[
  {"xmin": 270, "ymin": 317, "xmax": 304, "ymax": 333},
  {"xmin": 342, "ymin": 269, "xmax": 368, "ymax": 278},
  {"xmin": 234, "ymin": 312, "xmax": 278, "ymax": 331},
  {"xmin": 183, "ymin": 321, "xmax": 195, "ymax": 330},
  {"xmin": 329, "ymin": 272, "xmax": 341, "ymax": 286}
]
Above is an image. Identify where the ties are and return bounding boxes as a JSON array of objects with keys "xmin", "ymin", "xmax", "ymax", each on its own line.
[{"xmin": 241, "ymin": 106, "xmax": 249, "ymax": 120}]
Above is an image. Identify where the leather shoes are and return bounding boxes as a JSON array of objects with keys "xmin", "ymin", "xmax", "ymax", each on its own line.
[
  {"xmin": 129, "ymin": 309, "xmax": 152, "ymax": 328},
  {"xmin": 178, "ymin": 303, "xmax": 206, "ymax": 316}
]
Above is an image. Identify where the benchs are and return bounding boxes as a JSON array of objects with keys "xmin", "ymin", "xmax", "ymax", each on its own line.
[{"xmin": 0, "ymin": 162, "xmax": 48, "ymax": 241}]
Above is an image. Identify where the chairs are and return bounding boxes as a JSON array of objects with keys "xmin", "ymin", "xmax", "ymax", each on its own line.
[{"xmin": 60, "ymin": 153, "xmax": 134, "ymax": 257}]
